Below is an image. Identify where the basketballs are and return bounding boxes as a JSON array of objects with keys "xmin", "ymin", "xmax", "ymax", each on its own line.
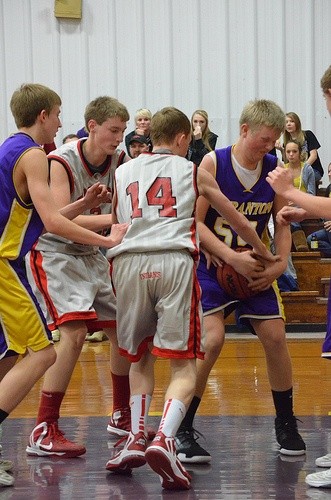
[{"xmin": 217, "ymin": 247, "xmax": 265, "ymax": 299}]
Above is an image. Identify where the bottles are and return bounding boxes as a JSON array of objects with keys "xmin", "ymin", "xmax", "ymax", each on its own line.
[
  {"xmin": 310, "ymin": 233, "xmax": 319, "ymax": 251},
  {"xmin": 320, "ymin": 217, "xmax": 326, "ymax": 227}
]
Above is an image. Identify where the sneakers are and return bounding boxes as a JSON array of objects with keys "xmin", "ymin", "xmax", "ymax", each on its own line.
[
  {"xmin": 25, "ymin": 421, "xmax": 86, "ymax": 458},
  {"xmin": 315, "ymin": 453, "xmax": 331, "ymax": 466},
  {"xmin": 305, "ymin": 469, "xmax": 331, "ymax": 488},
  {"xmin": 144, "ymin": 431, "xmax": 191, "ymax": 490},
  {"xmin": 51, "ymin": 329, "xmax": 60, "ymax": 341},
  {"xmin": 274, "ymin": 416, "xmax": 307, "ymax": 455},
  {"xmin": 107, "ymin": 409, "xmax": 156, "ymax": 440},
  {"xmin": 174, "ymin": 427, "xmax": 211, "ymax": 463},
  {"xmin": 85, "ymin": 331, "xmax": 108, "ymax": 342},
  {"xmin": 105, "ymin": 430, "xmax": 148, "ymax": 474},
  {"xmin": 0, "ymin": 446, "xmax": 15, "ymax": 486}
]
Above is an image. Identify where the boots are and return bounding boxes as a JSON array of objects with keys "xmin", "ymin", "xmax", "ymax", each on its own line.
[{"xmin": 292, "ymin": 230, "xmax": 309, "ymax": 252}]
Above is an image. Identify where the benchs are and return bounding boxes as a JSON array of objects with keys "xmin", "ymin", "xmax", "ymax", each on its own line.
[{"xmin": 222, "ymin": 218, "xmax": 331, "ymax": 323}]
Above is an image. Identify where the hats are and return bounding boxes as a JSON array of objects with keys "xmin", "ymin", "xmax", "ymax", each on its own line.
[{"xmin": 129, "ymin": 134, "xmax": 146, "ymax": 144}]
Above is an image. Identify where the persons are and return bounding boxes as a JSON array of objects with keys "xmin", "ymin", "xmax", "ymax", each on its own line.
[{"xmin": 0, "ymin": 65, "xmax": 331, "ymax": 489}]
